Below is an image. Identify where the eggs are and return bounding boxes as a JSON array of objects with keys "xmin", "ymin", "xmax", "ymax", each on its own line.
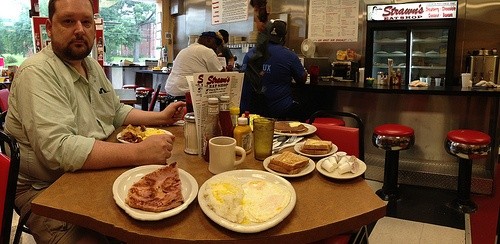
[
  {"xmin": 204, "ymin": 180, "xmax": 245, "ymax": 224},
  {"xmin": 243, "ymin": 180, "xmax": 291, "ymax": 222}
]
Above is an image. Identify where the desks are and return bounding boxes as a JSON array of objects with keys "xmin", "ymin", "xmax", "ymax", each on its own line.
[{"xmin": 133, "ymin": 69, "xmax": 500, "ymax": 177}]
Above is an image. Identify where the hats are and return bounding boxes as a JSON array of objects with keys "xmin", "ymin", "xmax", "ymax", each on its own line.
[{"xmin": 269, "ymin": 19, "xmax": 287, "ymax": 42}]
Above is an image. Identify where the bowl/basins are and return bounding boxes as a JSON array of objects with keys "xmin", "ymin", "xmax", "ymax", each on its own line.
[{"xmin": 365, "ymin": 78, "xmax": 374, "ymax": 84}]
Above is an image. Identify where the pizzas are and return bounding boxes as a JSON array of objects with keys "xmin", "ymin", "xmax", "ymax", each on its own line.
[{"xmin": 125, "ymin": 161, "xmax": 184, "ymax": 212}]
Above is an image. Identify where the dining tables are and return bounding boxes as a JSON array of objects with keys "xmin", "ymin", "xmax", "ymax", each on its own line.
[{"xmin": 31, "ymin": 124, "xmax": 388, "ymax": 244}]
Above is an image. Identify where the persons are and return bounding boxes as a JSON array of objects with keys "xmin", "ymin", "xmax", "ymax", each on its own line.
[
  {"xmin": 216, "ymin": 29, "xmax": 235, "ymax": 72},
  {"xmin": 165, "ymin": 31, "xmax": 224, "ymax": 102},
  {"xmin": 3, "ymin": 0, "xmax": 186, "ymax": 244},
  {"xmin": 240, "ymin": 19, "xmax": 308, "ymax": 119}
]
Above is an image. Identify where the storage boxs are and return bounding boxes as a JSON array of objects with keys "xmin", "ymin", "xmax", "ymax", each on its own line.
[
  {"xmin": 303, "ymin": 57, "xmax": 329, "ymax": 76},
  {"xmin": 331, "ymin": 61, "xmax": 362, "ymax": 80}
]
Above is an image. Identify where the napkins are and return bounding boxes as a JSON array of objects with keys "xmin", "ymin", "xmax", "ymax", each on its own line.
[{"xmin": 475, "ymin": 80, "xmax": 500, "ymax": 89}]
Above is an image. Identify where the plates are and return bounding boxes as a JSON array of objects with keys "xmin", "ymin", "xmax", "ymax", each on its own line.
[
  {"xmin": 293, "ymin": 141, "xmax": 338, "ymax": 157},
  {"xmin": 376, "ymin": 50, "xmax": 437, "ymax": 54},
  {"xmin": 273, "ymin": 123, "xmax": 317, "ymax": 136},
  {"xmin": 117, "ymin": 127, "xmax": 173, "ymax": 145},
  {"xmin": 112, "ymin": 165, "xmax": 198, "ymax": 220},
  {"xmin": 316, "ymin": 156, "xmax": 367, "ymax": 179},
  {"xmin": 198, "ymin": 169, "xmax": 297, "ymax": 233},
  {"xmin": 263, "ymin": 154, "xmax": 315, "ymax": 177},
  {"xmin": 152, "ymin": 69, "xmax": 163, "ymax": 72}
]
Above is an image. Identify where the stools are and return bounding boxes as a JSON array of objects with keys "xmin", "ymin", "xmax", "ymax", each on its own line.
[
  {"xmin": 373, "ymin": 124, "xmax": 415, "ymax": 201},
  {"xmin": 444, "ymin": 129, "xmax": 492, "ymax": 215}
]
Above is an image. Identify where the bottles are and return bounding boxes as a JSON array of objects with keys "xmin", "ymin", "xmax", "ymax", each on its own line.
[
  {"xmin": 163, "ymin": 51, "xmax": 167, "ymax": 62},
  {"xmin": 390, "ymin": 68, "xmax": 401, "ymax": 87},
  {"xmin": 202, "ymin": 98, "xmax": 220, "ymax": 162},
  {"xmin": 218, "ymin": 96, "xmax": 252, "ymax": 155},
  {"xmin": 184, "ymin": 113, "xmax": 198, "ymax": 155},
  {"xmin": 376, "ymin": 72, "xmax": 383, "ymax": 85}
]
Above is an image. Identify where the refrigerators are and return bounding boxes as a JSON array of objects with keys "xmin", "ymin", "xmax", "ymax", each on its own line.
[{"xmin": 364, "ymin": 1, "xmax": 459, "ymax": 86}]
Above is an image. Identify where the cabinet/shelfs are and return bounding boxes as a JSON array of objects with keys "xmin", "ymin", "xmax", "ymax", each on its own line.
[{"xmin": 364, "ymin": 0, "xmax": 458, "ymax": 94}]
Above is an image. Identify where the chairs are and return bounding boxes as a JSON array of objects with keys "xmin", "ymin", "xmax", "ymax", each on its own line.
[
  {"xmin": 307, "ymin": 109, "xmax": 366, "ymax": 244},
  {"xmin": 0, "ymin": 88, "xmax": 33, "ymax": 244}
]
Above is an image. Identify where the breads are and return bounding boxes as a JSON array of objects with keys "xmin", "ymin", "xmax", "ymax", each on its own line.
[
  {"xmin": 300, "ymin": 138, "xmax": 332, "ymax": 155},
  {"xmin": 267, "ymin": 151, "xmax": 309, "ymax": 174},
  {"xmin": 274, "ymin": 121, "xmax": 307, "ymax": 133}
]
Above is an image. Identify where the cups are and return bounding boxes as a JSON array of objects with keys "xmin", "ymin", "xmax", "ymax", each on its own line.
[
  {"xmin": 253, "ymin": 117, "xmax": 275, "ymax": 161},
  {"xmin": 309, "ymin": 66, "xmax": 319, "ymax": 84},
  {"xmin": 208, "ymin": 136, "xmax": 246, "ymax": 174},
  {"xmin": 419, "ymin": 77, "xmax": 441, "ymax": 86},
  {"xmin": 461, "ymin": 72, "xmax": 472, "ymax": 88},
  {"xmin": 157, "ymin": 59, "xmax": 162, "ymax": 67}
]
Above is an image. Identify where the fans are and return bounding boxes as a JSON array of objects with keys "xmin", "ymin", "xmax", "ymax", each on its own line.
[{"xmin": 300, "ymin": 39, "xmax": 316, "ymax": 58}]
